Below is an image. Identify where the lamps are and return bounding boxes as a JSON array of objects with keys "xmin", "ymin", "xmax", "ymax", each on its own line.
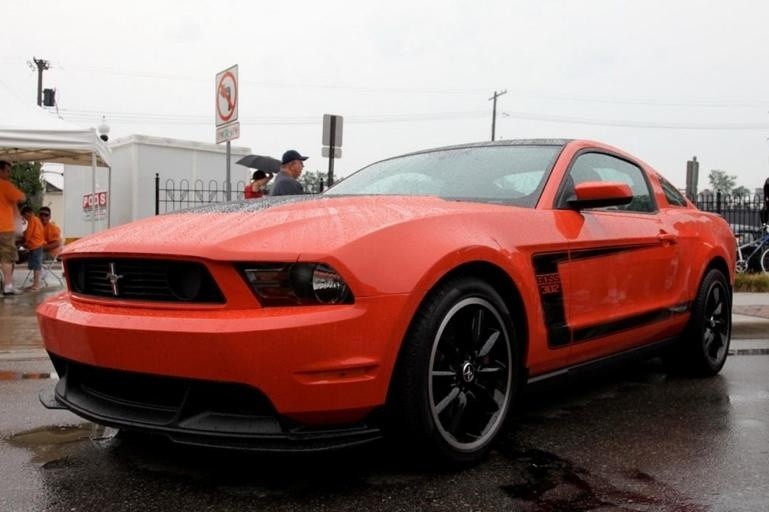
[{"xmin": 20, "ymin": 235, "xmax": 66, "ymax": 291}]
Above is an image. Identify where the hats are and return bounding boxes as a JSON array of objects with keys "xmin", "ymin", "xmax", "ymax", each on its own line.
[{"xmin": 34, "ymin": 138, "xmax": 736, "ymax": 466}]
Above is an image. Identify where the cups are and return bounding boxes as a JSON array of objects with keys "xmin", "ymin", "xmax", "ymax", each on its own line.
[{"xmin": 39, "ymin": 212, "xmax": 50, "ymax": 217}]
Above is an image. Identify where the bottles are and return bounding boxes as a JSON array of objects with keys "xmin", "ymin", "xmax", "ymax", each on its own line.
[{"xmin": 282, "ymin": 150, "xmax": 310, "ymax": 165}]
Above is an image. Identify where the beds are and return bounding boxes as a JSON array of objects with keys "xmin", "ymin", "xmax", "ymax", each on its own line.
[{"xmin": 234, "ymin": 155, "xmax": 282, "ymax": 180}]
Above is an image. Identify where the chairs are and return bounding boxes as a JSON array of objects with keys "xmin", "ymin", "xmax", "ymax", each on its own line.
[{"xmin": 3, "ymin": 284, "xmax": 41, "ymax": 296}]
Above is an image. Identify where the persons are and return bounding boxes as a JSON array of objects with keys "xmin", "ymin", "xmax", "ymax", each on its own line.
[
  {"xmin": 21, "ymin": 206, "xmax": 44, "ymax": 293},
  {"xmin": 245, "ymin": 169, "xmax": 273, "ymax": 198},
  {"xmin": 36, "ymin": 207, "xmax": 62, "ymax": 261},
  {"xmin": 270, "ymin": 151, "xmax": 309, "ymax": 196},
  {"xmin": 0, "ymin": 160, "xmax": 29, "ymax": 298}
]
